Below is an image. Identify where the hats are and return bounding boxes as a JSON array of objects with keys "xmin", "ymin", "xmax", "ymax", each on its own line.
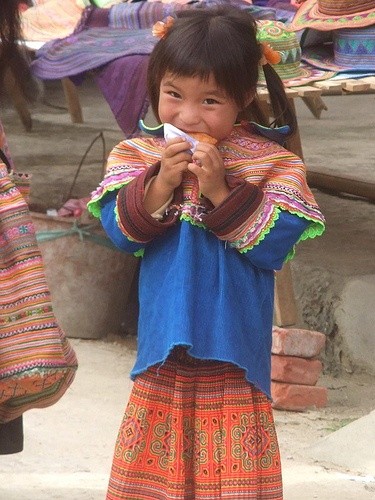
[
  {"xmin": 253, "ymin": 20, "xmax": 337, "ymax": 88},
  {"xmin": 288, "ymin": 0, "xmax": 375, "ymax": 30},
  {"xmin": 300, "ymin": 26, "xmax": 375, "ymax": 72}
]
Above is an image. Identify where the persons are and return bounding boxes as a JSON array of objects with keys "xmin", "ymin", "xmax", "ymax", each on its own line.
[
  {"xmin": 1, "ymin": 0, "xmax": 43, "ymax": 456},
  {"xmin": 85, "ymin": 6, "xmax": 327, "ymax": 500}
]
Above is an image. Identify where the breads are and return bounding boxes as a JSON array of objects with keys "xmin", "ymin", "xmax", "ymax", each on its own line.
[{"xmin": 187, "ymin": 133, "xmax": 219, "ymax": 148}]
